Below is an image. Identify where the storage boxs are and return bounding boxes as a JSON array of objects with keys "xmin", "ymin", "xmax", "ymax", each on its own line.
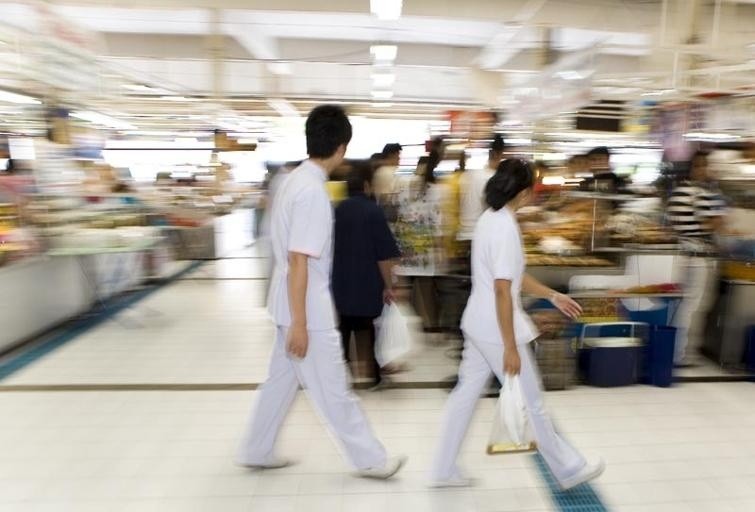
[{"xmin": 578, "ymin": 322, "xmax": 649, "ymax": 388}]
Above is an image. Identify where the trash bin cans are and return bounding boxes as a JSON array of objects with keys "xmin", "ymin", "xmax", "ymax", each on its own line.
[
  {"xmin": 535, "ymin": 337, "xmax": 576, "ymax": 391},
  {"xmin": 702, "ymin": 278, "xmax": 755, "ymax": 367}
]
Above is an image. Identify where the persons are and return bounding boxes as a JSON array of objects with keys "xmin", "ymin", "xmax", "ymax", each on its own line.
[
  {"xmin": 423, "ymin": 157, "xmax": 606, "ymax": 492},
  {"xmin": 249, "ymin": 132, "xmax": 755, "ymax": 386},
  {"xmin": 237, "ymin": 102, "xmax": 408, "ymax": 479}
]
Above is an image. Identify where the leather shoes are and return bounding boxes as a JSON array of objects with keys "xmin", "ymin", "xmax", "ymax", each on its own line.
[
  {"xmin": 560, "ymin": 453, "xmax": 606, "ymax": 492},
  {"xmin": 241, "ymin": 450, "xmax": 293, "ymax": 466},
  {"xmin": 432, "ymin": 475, "xmax": 472, "ymax": 488},
  {"xmin": 358, "ymin": 458, "xmax": 406, "ymax": 482}
]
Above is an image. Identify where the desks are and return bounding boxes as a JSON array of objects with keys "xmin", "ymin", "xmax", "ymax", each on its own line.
[
  {"xmin": 0, "ymin": 251, "xmax": 144, "ymax": 355},
  {"xmin": 164, "ymin": 208, "xmax": 256, "ymax": 261}
]
